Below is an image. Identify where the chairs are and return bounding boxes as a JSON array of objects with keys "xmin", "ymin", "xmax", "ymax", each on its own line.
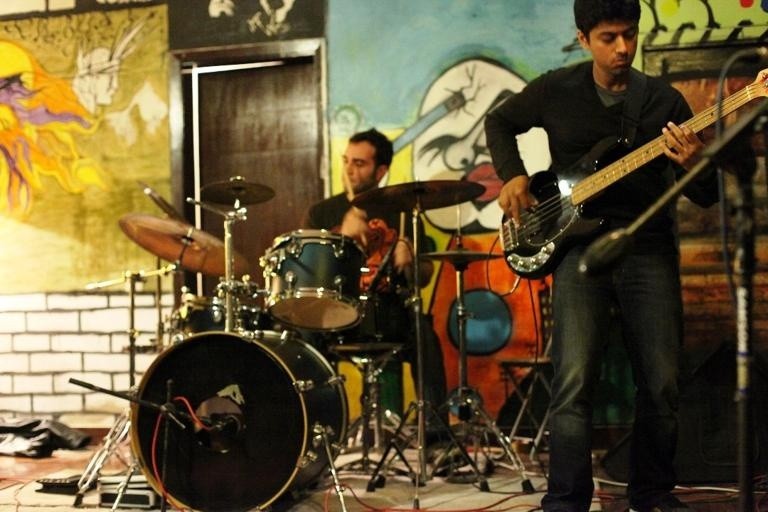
[{"xmin": 495, "ymin": 336, "xmax": 560, "ymax": 472}]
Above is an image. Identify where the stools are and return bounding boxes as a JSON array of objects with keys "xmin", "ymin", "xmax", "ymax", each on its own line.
[{"xmin": 324, "ymin": 341, "xmax": 411, "ymax": 486}]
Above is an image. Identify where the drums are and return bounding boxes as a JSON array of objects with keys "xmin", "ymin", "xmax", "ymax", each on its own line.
[
  {"xmin": 170, "ymin": 294, "xmax": 271, "ymax": 343},
  {"xmin": 267, "ymin": 230, "xmax": 364, "ymax": 330},
  {"xmin": 129, "ymin": 330, "xmax": 350, "ymax": 511}
]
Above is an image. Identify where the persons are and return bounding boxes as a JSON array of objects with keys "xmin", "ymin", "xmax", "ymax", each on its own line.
[
  {"xmin": 485, "ymin": 0, "xmax": 720, "ymax": 512},
  {"xmin": 288, "ymin": 127, "xmax": 469, "ymax": 472}
]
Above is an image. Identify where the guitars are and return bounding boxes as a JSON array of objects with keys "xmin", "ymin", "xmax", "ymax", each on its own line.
[{"xmin": 500, "ymin": 70, "xmax": 768, "ymax": 280}]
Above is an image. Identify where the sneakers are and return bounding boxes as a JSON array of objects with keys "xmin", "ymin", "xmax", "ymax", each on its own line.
[{"xmin": 427, "ymin": 449, "xmax": 468, "ymax": 473}]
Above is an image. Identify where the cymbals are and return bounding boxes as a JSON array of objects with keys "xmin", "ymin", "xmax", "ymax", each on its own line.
[
  {"xmin": 423, "ymin": 249, "xmax": 500, "ymax": 264},
  {"xmin": 199, "ymin": 181, "xmax": 274, "ymax": 204},
  {"xmin": 137, "ymin": 180, "xmax": 183, "ymax": 219},
  {"xmin": 350, "ymin": 180, "xmax": 485, "ymax": 214},
  {"xmin": 118, "ymin": 213, "xmax": 251, "ymax": 277}
]
